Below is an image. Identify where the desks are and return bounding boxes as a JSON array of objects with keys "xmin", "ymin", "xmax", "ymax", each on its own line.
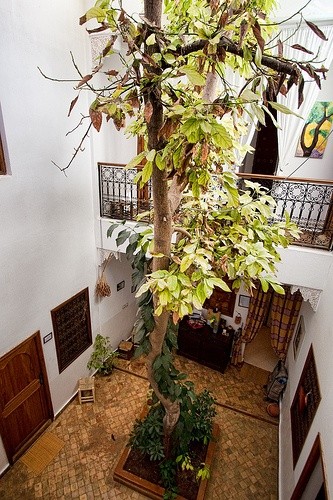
[{"xmin": 78, "ymin": 376, "xmax": 96, "ymax": 403}]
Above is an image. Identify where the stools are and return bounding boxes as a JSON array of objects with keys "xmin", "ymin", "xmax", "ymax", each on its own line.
[{"xmin": 117, "ymin": 339, "xmax": 133, "ymax": 361}]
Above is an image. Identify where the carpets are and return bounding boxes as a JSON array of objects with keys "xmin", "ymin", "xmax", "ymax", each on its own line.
[{"xmin": 19, "ymin": 432, "xmax": 65, "ymax": 476}]
